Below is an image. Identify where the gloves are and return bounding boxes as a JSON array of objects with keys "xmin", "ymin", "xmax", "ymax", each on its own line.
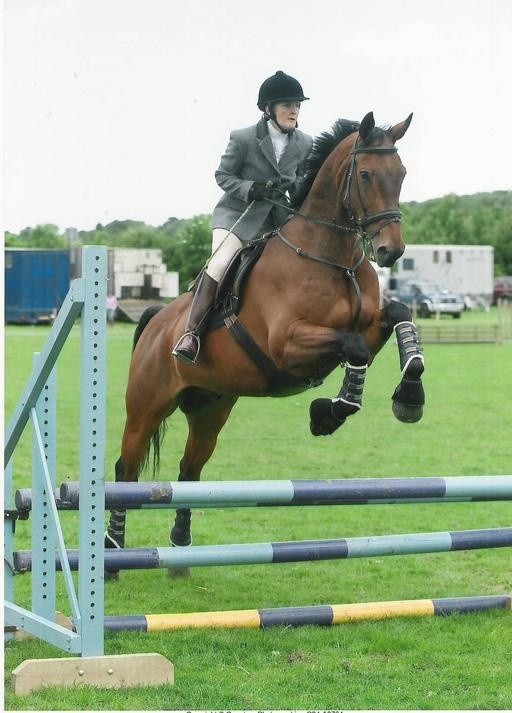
[{"xmin": 249, "ymin": 181, "xmax": 271, "ymax": 200}]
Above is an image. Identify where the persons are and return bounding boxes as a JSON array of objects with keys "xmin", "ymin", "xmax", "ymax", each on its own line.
[
  {"xmin": 173, "ymin": 70, "xmax": 315, "ymax": 363},
  {"xmin": 105, "ymin": 292, "xmax": 119, "ymax": 326}
]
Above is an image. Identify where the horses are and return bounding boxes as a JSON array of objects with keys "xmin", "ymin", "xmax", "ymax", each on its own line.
[{"xmin": 102, "ymin": 110, "xmax": 426, "ymax": 586}]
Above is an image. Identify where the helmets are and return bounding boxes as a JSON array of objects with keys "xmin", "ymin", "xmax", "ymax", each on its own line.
[{"xmin": 258, "ymin": 71, "xmax": 309, "ymax": 104}]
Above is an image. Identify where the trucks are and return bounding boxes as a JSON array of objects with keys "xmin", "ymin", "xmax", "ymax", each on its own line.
[{"xmin": 368, "ymin": 244, "xmax": 495, "ymax": 308}]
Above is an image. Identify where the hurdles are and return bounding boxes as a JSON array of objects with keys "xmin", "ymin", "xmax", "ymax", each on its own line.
[{"xmin": 5, "ymin": 244, "xmax": 512, "ymax": 695}]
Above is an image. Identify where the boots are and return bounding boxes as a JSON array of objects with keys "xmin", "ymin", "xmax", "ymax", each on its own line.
[{"xmin": 176, "ymin": 270, "xmax": 219, "ymax": 360}]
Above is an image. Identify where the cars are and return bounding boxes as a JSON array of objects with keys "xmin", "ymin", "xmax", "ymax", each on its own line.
[{"xmin": 491, "ymin": 274, "xmax": 512, "ymax": 301}]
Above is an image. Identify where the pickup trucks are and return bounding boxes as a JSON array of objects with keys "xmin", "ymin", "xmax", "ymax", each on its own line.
[{"xmin": 383, "ymin": 278, "xmax": 466, "ymax": 319}]
